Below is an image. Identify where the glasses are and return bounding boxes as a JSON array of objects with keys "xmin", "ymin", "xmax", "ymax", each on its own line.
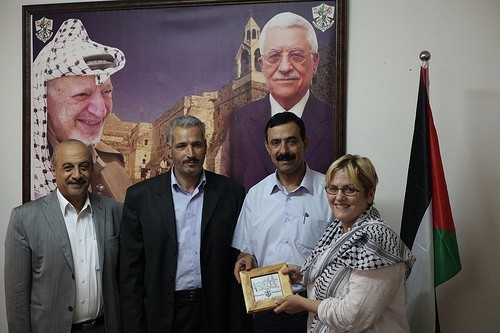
[
  {"xmin": 325, "ymin": 186, "xmax": 359, "ymax": 195},
  {"xmin": 261, "ymin": 48, "xmax": 314, "ymax": 63}
]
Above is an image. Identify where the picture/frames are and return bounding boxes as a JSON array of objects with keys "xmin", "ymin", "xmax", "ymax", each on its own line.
[
  {"xmin": 238, "ymin": 262, "xmax": 294, "ymax": 314},
  {"xmin": 21, "ymin": 1, "xmax": 347, "ymax": 205}
]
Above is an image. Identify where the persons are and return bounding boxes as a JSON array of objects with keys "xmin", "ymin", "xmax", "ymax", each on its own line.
[
  {"xmin": 4, "ymin": 139, "xmax": 124, "ymax": 333},
  {"xmin": 119, "ymin": 114, "xmax": 253, "ymax": 333},
  {"xmin": 231, "ymin": 111, "xmax": 336, "ymax": 333},
  {"xmin": 223, "ymin": 11, "xmax": 335, "ymax": 193},
  {"xmin": 31, "ymin": 18, "xmax": 134, "ymax": 204},
  {"xmin": 273, "ymin": 154, "xmax": 417, "ymax": 333}
]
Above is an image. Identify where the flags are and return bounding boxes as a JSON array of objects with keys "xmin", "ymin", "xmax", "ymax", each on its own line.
[{"xmin": 400, "ymin": 61, "xmax": 464, "ymax": 333}]
